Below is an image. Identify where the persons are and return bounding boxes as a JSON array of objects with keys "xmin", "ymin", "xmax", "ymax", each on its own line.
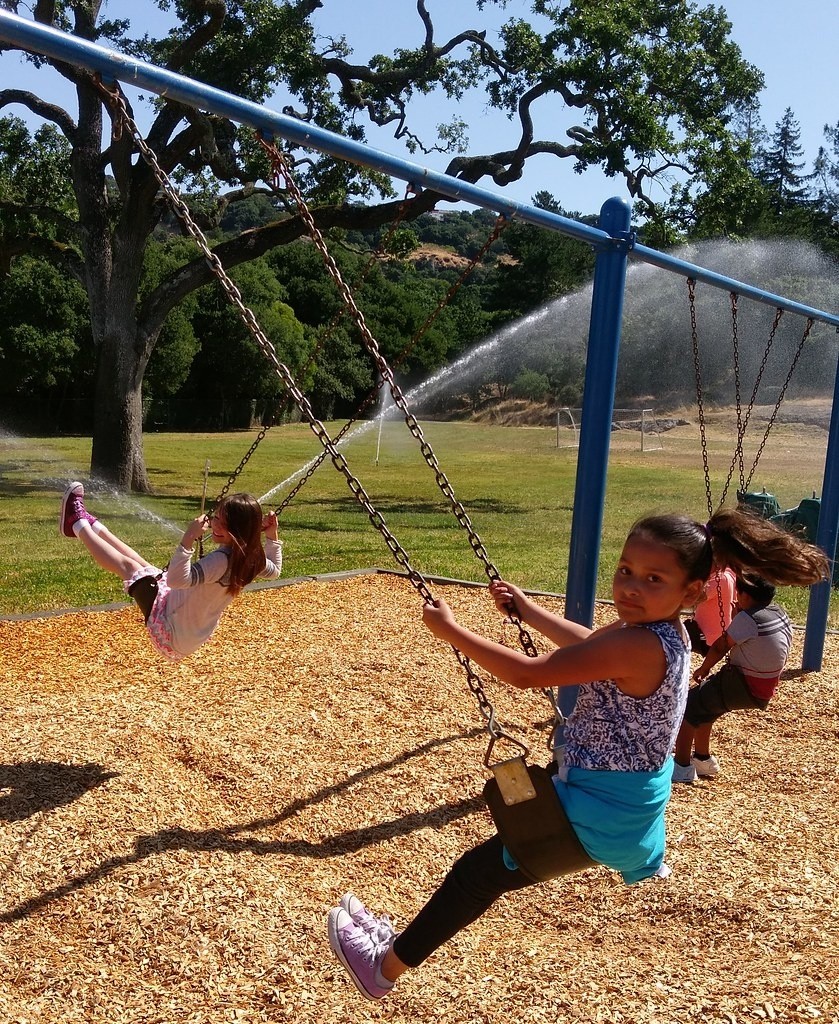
[
  {"xmin": 60, "ymin": 480, "xmax": 282, "ymax": 660},
  {"xmin": 671, "ymin": 574, "xmax": 792, "ymax": 783},
  {"xmin": 328, "ymin": 510, "xmax": 828, "ymax": 1001},
  {"xmin": 684, "ymin": 558, "xmax": 737, "ymax": 657}
]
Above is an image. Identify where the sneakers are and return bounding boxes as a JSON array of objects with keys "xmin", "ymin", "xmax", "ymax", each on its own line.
[
  {"xmin": 671, "ymin": 754, "xmax": 722, "ymax": 783},
  {"xmin": 60, "ymin": 481, "xmax": 99, "ymax": 539},
  {"xmin": 328, "ymin": 894, "xmax": 405, "ymax": 1001}
]
